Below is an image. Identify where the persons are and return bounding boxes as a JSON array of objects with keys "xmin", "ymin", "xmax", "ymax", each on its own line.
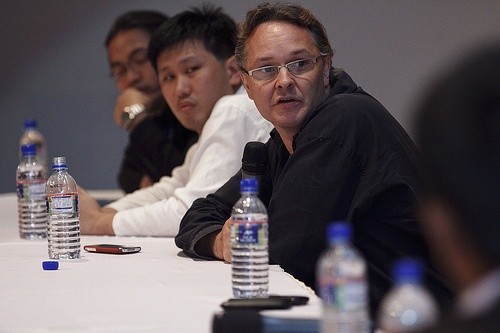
[
  {"xmin": 103, "ymin": 9, "xmax": 199, "ymax": 195},
  {"xmin": 175, "ymin": 2, "xmax": 455, "ymax": 333},
  {"xmin": 413, "ymin": 34, "xmax": 500, "ymax": 333},
  {"xmin": 76, "ymin": 3, "xmax": 276, "ymax": 236}
]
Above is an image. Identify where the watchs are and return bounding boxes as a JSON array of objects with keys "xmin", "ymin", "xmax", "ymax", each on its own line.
[{"xmin": 118, "ymin": 104, "xmax": 147, "ymax": 130}]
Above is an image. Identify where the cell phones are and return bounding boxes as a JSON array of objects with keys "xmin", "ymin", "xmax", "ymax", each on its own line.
[{"xmin": 83, "ymin": 245, "xmax": 141, "ymax": 253}]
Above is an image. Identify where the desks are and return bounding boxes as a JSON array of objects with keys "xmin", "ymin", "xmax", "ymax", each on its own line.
[{"xmin": 0, "ymin": 191, "xmax": 324, "ymax": 333}]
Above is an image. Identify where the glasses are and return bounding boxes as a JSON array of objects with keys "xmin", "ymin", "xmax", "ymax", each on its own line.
[
  {"xmin": 108, "ymin": 48, "xmax": 150, "ymax": 76},
  {"xmin": 236, "ymin": 55, "xmax": 324, "ymax": 80}
]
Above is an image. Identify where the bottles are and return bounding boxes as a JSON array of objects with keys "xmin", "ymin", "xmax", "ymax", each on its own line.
[
  {"xmin": 230, "ymin": 178, "xmax": 270, "ymax": 299},
  {"xmin": 16, "ymin": 144, "xmax": 47, "ymax": 240},
  {"xmin": 19, "ymin": 119, "xmax": 47, "ymax": 172},
  {"xmin": 315, "ymin": 222, "xmax": 370, "ymax": 333},
  {"xmin": 45, "ymin": 156, "xmax": 80, "ymax": 261},
  {"xmin": 377, "ymin": 258, "xmax": 440, "ymax": 333}
]
